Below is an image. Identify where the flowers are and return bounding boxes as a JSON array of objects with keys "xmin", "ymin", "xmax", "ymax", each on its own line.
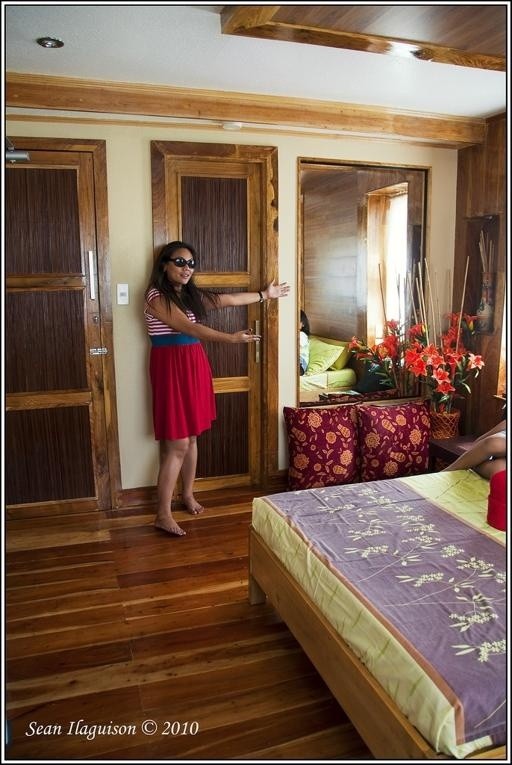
[
  {"xmin": 405, "ymin": 306, "xmax": 486, "ymax": 412},
  {"xmin": 346, "ymin": 317, "xmax": 421, "ymax": 388}
]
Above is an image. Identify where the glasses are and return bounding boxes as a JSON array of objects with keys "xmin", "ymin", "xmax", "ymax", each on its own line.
[{"xmin": 169, "ymin": 255, "xmax": 195, "ymax": 269}]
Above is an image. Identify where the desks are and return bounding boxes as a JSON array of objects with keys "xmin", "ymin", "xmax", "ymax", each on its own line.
[{"xmin": 429, "ymin": 432, "xmax": 480, "ymax": 473}]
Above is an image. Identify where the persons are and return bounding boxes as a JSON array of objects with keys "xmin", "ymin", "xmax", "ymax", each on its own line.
[
  {"xmin": 436, "ymin": 418, "xmax": 507, "ymax": 480},
  {"xmin": 142, "ymin": 241, "xmax": 291, "ymax": 536},
  {"xmin": 300, "ymin": 309, "xmax": 309, "ymax": 375}
]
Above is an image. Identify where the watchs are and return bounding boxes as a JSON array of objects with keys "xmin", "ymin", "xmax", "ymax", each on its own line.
[{"xmin": 257, "ymin": 290, "xmax": 264, "ymax": 305}]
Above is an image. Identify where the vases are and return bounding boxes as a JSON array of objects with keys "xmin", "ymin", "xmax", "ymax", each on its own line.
[
  {"xmin": 477, "ymin": 272, "xmax": 496, "ymax": 333},
  {"xmin": 432, "ymin": 408, "xmax": 461, "ymax": 441}
]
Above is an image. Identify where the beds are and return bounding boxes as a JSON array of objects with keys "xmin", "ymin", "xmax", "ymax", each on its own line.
[
  {"xmin": 300, "ymin": 368, "xmax": 357, "ymax": 403},
  {"xmin": 250, "ymin": 466, "xmax": 506, "ymax": 760}
]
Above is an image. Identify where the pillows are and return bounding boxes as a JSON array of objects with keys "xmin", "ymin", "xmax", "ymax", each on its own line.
[
  {"xmin": 305, "ymin": 336, "xmax": 345, "ymax": 375},
  {"xmin": 318, "ymin": 337, "xmax": 353, "ymax": 370},
  {"xmin": 280, "ymin": 403, "xmax": 360, "ymax": 490},
  {"xmin": 358, "ymin": 398, "xmax": 433, "ymax": 481}
]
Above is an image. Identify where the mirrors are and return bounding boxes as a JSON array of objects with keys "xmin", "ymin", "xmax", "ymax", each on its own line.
[{"xmin": 296, "ymin": 154, "xmax": 435, "ymax": 409}]
